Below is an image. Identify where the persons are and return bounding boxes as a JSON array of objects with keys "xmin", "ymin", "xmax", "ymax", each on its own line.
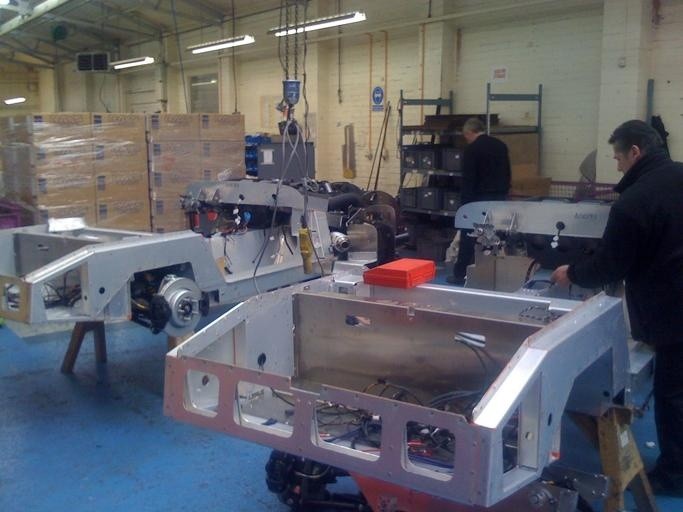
[
  {"xmin": 550, "ymin": 116, "xmax": 681, "ymax": 499},
  {"xmin": 444, "ymin": 121, "xmax": 510, "ymax": 285}
]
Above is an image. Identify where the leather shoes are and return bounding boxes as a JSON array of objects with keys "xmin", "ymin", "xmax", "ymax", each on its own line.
[
  {"xmin": 447, "ymin": 276, "xmax": 465, "ymax": 284},
  {"xmin": 627, "ymin": 473, "xmax": 680, "ymax": 497}
]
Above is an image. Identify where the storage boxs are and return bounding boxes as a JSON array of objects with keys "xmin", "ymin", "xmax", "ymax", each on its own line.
[
  {"xmin": 399, "ymin": 129, "xmax": 543, "ymax": 213},
  {"xmin": 0, "ymin": 111, "xmax": 248, "ymax": 233}
]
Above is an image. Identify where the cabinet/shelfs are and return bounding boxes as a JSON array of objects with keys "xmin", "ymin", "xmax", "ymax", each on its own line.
[{"xmin": 398, "ymin": 82, "xmax": 543, "ymax": 218}]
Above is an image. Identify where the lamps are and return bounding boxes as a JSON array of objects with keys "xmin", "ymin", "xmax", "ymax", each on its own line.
[
  {"xmin": 185, "ymin": 33, "xmax": 256, "ymax": 55},
  {"xmin": 266, "ymin": 11, "xmax": 366, "ymax": 36},
  {"xmin": 109, "ymin": 54, "xmax": 155, "ymax": 70}
]
